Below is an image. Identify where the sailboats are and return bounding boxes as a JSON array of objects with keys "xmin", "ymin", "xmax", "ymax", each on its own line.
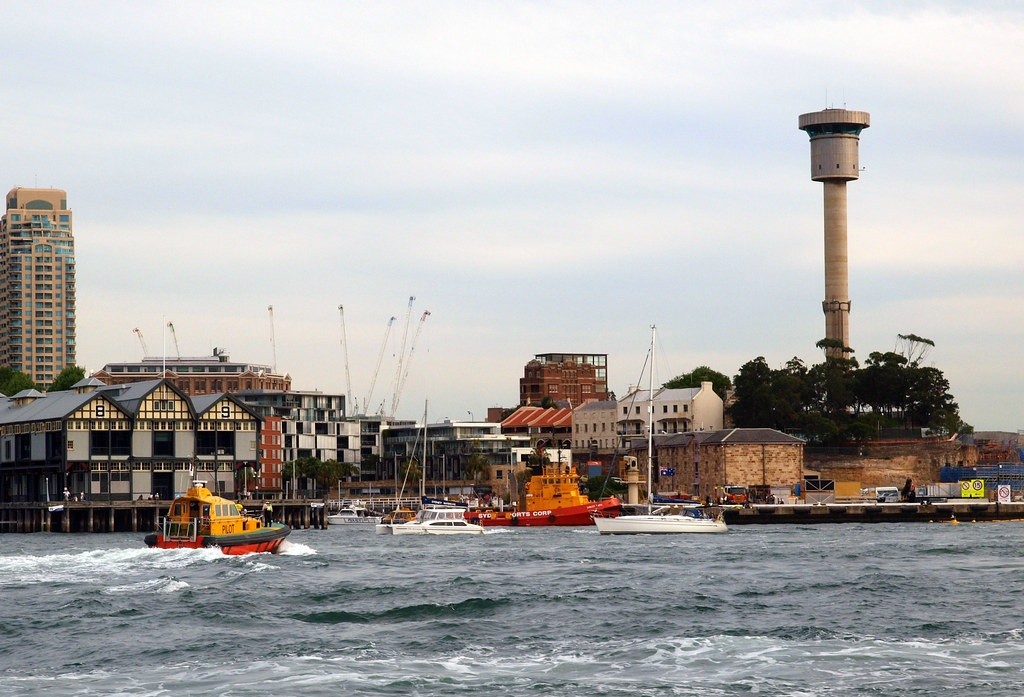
[
  {"xmin": 586, "ymin": 322, "xmax": 729, "ymax": 535},
  {"xmin": 374, "ymin": 398, "xmax": 486, "ymax": 536}
]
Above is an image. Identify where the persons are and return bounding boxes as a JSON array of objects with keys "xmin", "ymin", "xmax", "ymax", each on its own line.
[
  {"xmin": 263, "ymin": 501, "xmax": 273, "ymax": 527},
  {"xmin": 73, "ymin": 490, "xmax": 84, "ymax": 502},
  {"xmin": 901, "ymin": 477, "xmax": 912, "ymax": 499},
  {"xmin": 148, "ymin": 492, "xmax": 159, "ymax": 500},
  {"xmin": 235, "ymin": 500, "xmax": 243, "ymax": 514}
]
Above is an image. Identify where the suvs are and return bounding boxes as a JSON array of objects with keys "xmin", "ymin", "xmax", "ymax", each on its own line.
[{"xmin": 722, "ymin": 485, "xmax": 749, "ymax": 505}]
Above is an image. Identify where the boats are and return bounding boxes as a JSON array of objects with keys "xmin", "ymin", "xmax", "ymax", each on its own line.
[
  {"xmin": 461, "ymin": 439, "xmax": 622, "ymax": 528},
  {"xmin": 381, "ymin": 506, "xmax": 417, "ymax": 524},
  {"xmin": 327, "ymin": 505, "xmax": 384, "ymax": 524},
  {"xmin": 142, "ymin": 473, "xmax": 291, "ymax": 556}
]
